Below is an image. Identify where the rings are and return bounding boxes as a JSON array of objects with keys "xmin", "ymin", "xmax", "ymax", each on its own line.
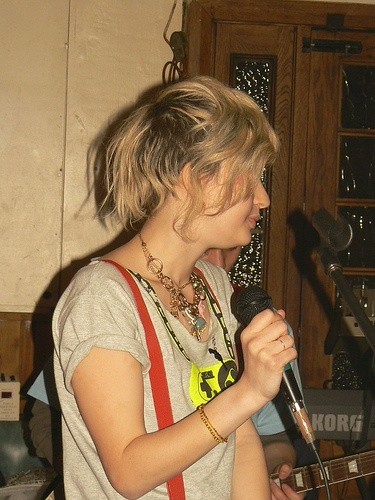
[{"xmin": 279, "ymin": 339, "xmax": 285, "ymax": 350}]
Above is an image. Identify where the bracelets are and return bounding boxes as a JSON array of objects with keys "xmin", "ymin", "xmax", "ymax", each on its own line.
[{"xmin": 199, "ymin": 405, "xmax": 228, "ymax": 443}]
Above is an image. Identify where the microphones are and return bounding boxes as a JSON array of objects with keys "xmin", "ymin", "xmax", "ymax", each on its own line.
[
  {"xmin": 312, "ymin": 208, "xmax": 353, "ymax": 251},
  {"xmin": 234, "ymin": 285, "xmax": 317, "ymax": 452}
]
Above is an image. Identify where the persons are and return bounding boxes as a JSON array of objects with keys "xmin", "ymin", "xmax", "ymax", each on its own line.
[
  {"xmin": 27, "ymin": 247, "xmax": 307, "ymax": 500},
  {"xmin": 51, "ymin": 75, "xmax": 299, "ymax": 500}
]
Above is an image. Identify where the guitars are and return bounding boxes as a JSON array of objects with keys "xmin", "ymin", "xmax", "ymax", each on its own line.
[{"xmin": 0, "ymin": 450, "xmax": 375, "ymax": 500}]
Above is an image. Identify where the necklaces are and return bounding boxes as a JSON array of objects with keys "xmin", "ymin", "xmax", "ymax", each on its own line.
[{"xmin": 137, "ymin": 232, "xmax": 206, "ymax": 341}]
